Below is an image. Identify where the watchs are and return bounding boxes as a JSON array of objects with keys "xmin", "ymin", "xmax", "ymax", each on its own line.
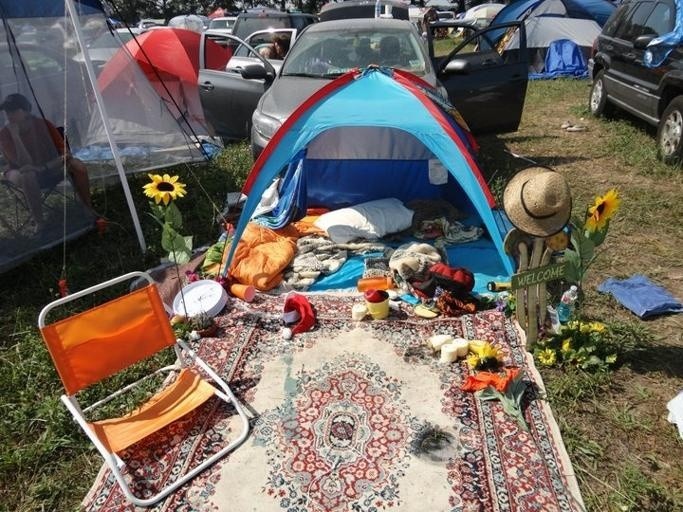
[{"xmin": 41, "ymin": 162, "xmax": 49, "ymax": 171}]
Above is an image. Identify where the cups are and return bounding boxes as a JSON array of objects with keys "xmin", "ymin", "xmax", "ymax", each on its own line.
[{"xmin": 365, "ymin": 293, "xmax": 389, "ymax": 320}]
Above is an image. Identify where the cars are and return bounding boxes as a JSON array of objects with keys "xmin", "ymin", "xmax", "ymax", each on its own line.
[
  {"xmin": 207, "ymin": 16, "xmax": 237, "ymax": 48},
  {"xmin": 196, "ymin": 20, "xmax": 529, "ymax": 165},
  {"xmin": 69, "ymin": 28, "xmax": 147, "ymax": 71}
]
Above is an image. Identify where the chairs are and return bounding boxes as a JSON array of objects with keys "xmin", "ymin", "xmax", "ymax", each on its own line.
[
  {"xmin": 2, "ymin": 125, "xmax": 80, "ymax": 229},
  {"xmin": 37, "ymin": 271, "xmax": 250, "ymax": 507}
]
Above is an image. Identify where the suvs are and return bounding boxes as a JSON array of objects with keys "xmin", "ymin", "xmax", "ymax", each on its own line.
[{"xmin": 587, "ymin": 1, "xmax": 683, "ymax": 165}]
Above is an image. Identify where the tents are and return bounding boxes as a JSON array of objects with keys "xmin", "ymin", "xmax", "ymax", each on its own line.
[
  {"xmin": 78, "ymin": 25, "xmax": 237, "ymax": 147},
  {"xmin": 0, "ymin": 0, "xmax": 208, "ymax": 269},
  {"xmin": 476, "ymin": 0, "xmax": 623, "ymax": 82},
  {"xmin": 460, "ymin": 3, "xmax": 506, "ymax": 38},
  {"xmin": 218, "ymin": 63, "xmax": 542, "ymax": 308}
]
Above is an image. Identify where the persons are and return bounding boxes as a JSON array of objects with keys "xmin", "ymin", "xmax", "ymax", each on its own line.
[
  {"xmin": 0, "ymin": 94, "xmax": 97, "ymax": 238},
  {"xmin": 247, "ymin": 35, "xmax": 291, "ymax": 60}
]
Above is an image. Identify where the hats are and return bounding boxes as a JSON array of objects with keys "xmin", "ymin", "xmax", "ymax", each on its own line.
[
  {"xmin": 280, "ymin": 292, "xmax": 317, "ymax": 341},
  {"xmin": 0, "ymin": 93, "xmax": 32, "ymax": 113},
  {"xmin": 502, "ymin": 164, "xmax": 573, "ymax": 238}
]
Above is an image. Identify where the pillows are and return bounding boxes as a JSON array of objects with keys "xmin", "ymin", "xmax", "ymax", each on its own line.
[{"xmin": 314, "ymin": 197, "xmax": 414, "ymax": 245}]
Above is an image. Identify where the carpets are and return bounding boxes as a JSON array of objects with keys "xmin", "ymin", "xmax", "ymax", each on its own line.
[{"xmin": 76, "ymin": 290, "xmax": 587, "ymax": 511}]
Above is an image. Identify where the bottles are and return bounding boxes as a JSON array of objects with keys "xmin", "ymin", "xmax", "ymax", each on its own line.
[{"xmin": 558, "ymin": 285, "xmax": 578, "ymax": 323}]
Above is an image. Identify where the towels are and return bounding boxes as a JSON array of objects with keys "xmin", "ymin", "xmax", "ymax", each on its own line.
[{"xmin": 596, "ymin": 270, "xmax": 682, "ymax": 319}]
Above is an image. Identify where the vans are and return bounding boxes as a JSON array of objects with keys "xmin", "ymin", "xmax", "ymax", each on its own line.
[
  {"xmin": 227, "ymin": 11, "xmax": 319, "ymax": 54},
  {"xmin": 434, "ymin": 9, "xmax": 457, "ymax": 24}
]
